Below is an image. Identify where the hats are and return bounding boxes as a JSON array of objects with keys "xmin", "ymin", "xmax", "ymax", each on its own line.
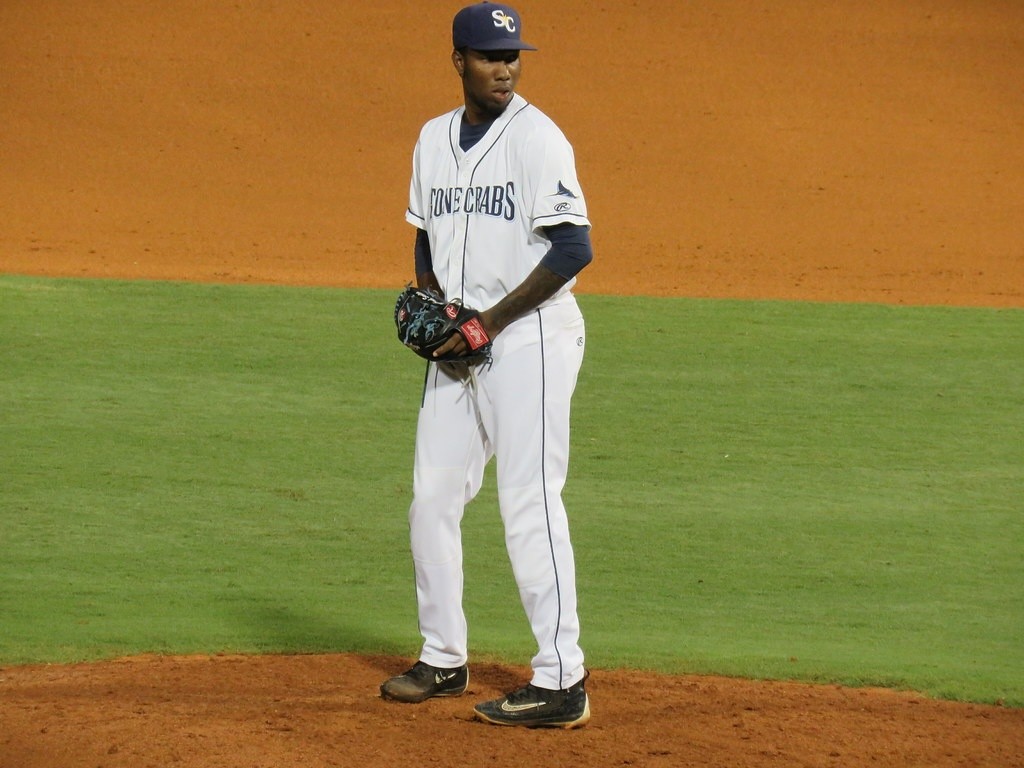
[{"xmin": 452, "ymin": 1, "xmax": 538, "ymax": 51}]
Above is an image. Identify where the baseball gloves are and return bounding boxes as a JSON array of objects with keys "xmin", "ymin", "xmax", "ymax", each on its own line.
[{"xmin": 393, "ymin": 279, "xmax": 494, "ymax": 409}]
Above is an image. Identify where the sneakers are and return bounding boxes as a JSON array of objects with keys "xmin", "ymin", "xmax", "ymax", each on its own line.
[
  {"xmin": 473, "ymin": 669, "xmax": 591, "ymax": 727},
  {"xmin": 380, "ymin": 661, "xmax": 469, "ymax": 703}
]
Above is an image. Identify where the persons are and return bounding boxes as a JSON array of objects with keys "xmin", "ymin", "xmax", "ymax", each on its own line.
[{"xmin": 379, "ymin": 1, "xmax": 594, "ymax": 730}]
